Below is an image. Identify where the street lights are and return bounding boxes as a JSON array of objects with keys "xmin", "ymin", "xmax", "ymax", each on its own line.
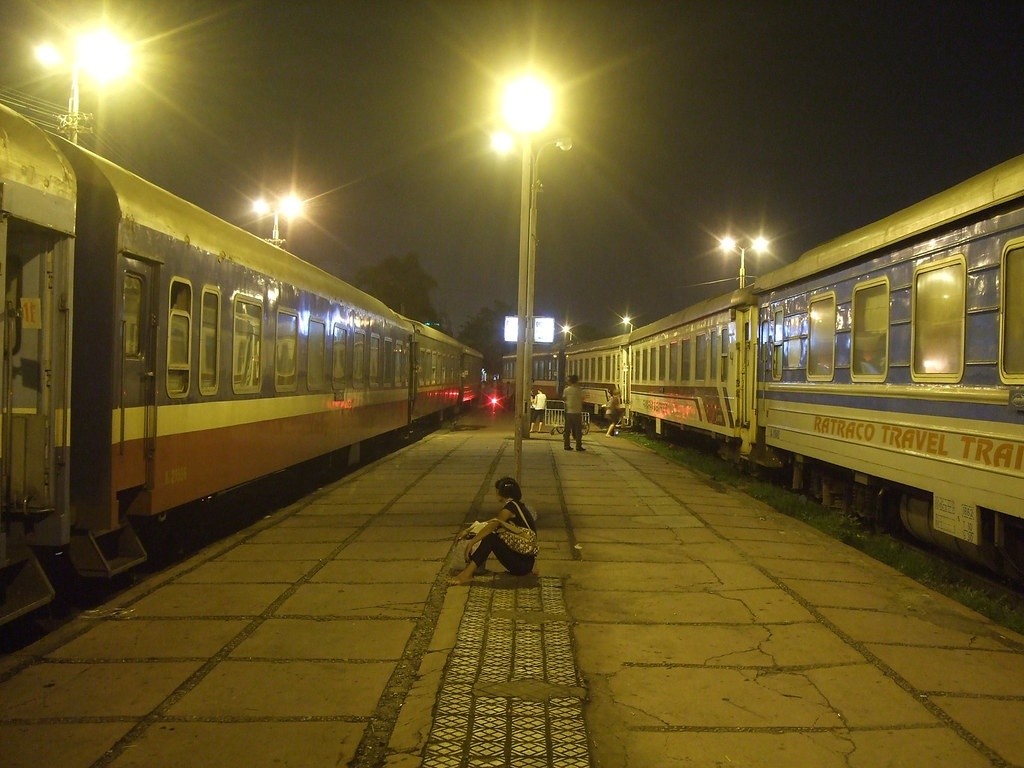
[
  {"xmin": 494, "ymin": 67, "xmax": 562, "ymax": 501},
  {"xmin": 722, "ymin": 235, "xmax": 768, "ymax": 289},
  {"xmin": 33, "ymin": 19, "xmax": 136, "ymax": 144},
  {"xmin": 251, "ymin": 193, "xmax": 303, "ymax": 249}
]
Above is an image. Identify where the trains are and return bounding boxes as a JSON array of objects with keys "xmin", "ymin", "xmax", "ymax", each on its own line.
[
  {"xmin": 0, "ymin": 102, "xmax": 484, "ymax": 625},
  {"xmin": 501, "ymin": 153, "xmax": 1024, "ymax": 586}
]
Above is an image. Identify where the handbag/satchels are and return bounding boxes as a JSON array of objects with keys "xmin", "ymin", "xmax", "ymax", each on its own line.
[{"xmin": 488, "ymin": 501, "xmax": 536, "ymax": 556}]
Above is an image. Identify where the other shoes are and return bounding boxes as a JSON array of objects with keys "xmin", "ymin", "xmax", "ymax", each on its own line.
[
  {"xmin": 564, "ymin": 447, "xmax": 573, "ymax": 450},
  {"xmin": 445, "ymin": 576, "xmax": 474, "ymax": 586},
  {"xmin": 576, "ymin": 447, "xmax": 585, "ymax": 451}
]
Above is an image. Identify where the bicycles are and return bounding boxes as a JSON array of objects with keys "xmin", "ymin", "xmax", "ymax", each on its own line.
[{"xmin": 551, "ymin": 410, "xmax": 590, "ymax": 436}]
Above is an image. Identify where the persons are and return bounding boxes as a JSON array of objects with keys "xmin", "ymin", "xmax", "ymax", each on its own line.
[
  {"xmin": 605, "ymin": 389, "xmax": 621, "ymax": 438},
  {"xmin": 446, "ymin": 477, "xmax": 540, "ymax": 586},
  {"xmin": 481, "ymin": 378, "xmax": 547, "ymax": 433},
  {"xmin": 562, "ymin": 374, "xmax": 587, "ymax": 451}
]
